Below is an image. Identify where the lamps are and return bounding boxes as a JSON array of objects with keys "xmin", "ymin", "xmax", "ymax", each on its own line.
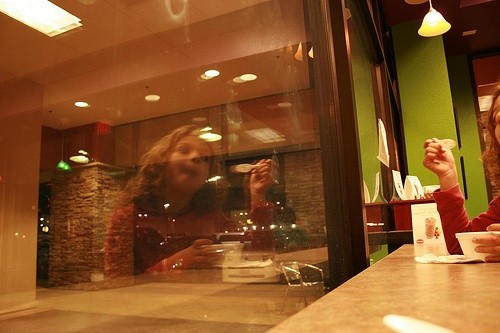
[{"xmin": 418, "ymin": 0, "xmax": 451, "ymax": 37}]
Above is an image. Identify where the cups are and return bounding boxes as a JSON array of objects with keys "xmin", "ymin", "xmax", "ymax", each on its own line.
[{"xmin": 425, "ymin": 218, "xmax": 436, "ymax": 239}]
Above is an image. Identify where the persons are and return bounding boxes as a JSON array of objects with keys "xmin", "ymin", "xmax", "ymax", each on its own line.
[
  {"xmin": 423, "ymin": 83, "xmax": 500, "ymax": 262},
  {"xmin": 103, "ymin": 123, "xmax": 278, "ymax": 278}
]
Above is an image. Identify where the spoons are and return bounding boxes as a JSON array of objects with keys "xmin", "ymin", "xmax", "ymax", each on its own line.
[
  {"xmin": 435, "ymin": 138, "xmax": 456, "ymax": 149},
  {"xmin": 234, "ymin": 163, "xmax": 259, "ymax": 173}
]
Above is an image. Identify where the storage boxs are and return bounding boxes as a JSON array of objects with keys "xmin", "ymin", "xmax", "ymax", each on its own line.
[{"xmin": 390, "ymin": 197, "xmax": 436, "ymax": 230}]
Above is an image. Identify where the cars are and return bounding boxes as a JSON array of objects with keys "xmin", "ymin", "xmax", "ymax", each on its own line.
[{"xmin": 135, "ymin": 229, "xmax": 300, "ymax": 283}]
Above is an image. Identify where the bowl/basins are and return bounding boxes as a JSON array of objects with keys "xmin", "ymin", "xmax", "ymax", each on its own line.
[
  {"xmin": 201, "ymin": 242, "xmax": 245, "ymax": 268},
  {"xmin": 455, "ymin": 232, "xmax": 500, "ymax": 259}
]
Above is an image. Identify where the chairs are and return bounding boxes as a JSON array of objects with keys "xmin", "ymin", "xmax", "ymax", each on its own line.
[{"xmin": 277, "ymin": 261, "xmax": 325, "ymax": 312}]
